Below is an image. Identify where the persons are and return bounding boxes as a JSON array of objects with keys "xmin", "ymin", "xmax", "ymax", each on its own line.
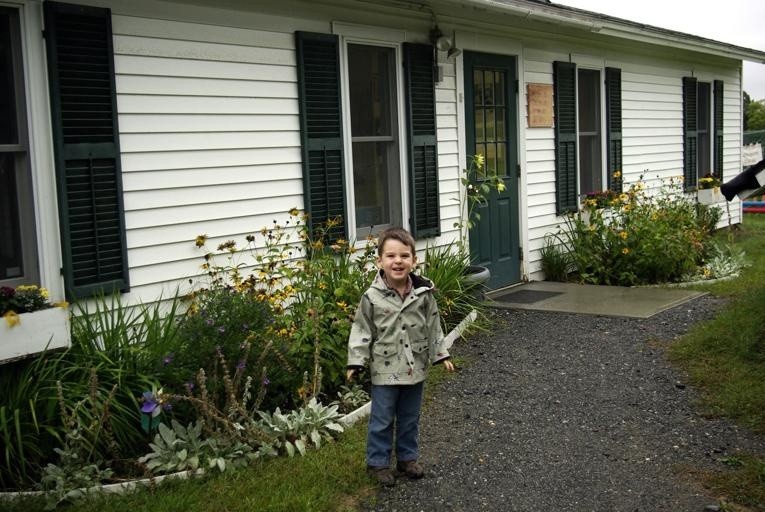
[{"xmin": 346, "ymin": 228, "xmax": 454, "ymax": 488}]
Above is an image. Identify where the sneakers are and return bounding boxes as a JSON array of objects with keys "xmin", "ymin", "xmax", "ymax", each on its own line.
[
  {"xmin": 366, "ymin": 466, "xmax": 395, "ymax": 487},
  {"xmin": 396, "ymin": 461, "xmax": 423, "ymax": 479}
]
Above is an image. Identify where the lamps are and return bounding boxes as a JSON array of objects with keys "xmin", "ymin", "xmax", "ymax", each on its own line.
[
  {"xmin": 429, "ymin": 11, "xmax": 452, "ymax": 82},
  {"xmin": 447, "ymin": 30, "xmax": 461, "ymax": 58}
]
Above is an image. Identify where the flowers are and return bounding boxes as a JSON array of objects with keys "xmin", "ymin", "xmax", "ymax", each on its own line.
[
  {"xmin": 698, "ymin": 173, "xmax": 720, "ymax": 182},
  {"xmin": 450, "ymin": 154, "xmax": 507, "ymax": 266},
  {"xmin": 0, "ymin": 285, "xmax": 70, "ymax": 326},
  {"xmin": 585, "ymin": 191, "xmax": 607, "ymax": 207}
]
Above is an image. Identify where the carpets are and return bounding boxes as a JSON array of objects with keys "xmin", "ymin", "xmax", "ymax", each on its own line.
[{"xmin": 486, "ymin": 289, "xmax": 565, "ymax": 304}]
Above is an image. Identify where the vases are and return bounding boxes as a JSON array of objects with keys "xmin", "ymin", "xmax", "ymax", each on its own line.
[
  {"xmin": 701, "ymin": 183, "xmax": 712, "ymax": 189},
  {"xmin": 596, "ymin": 204, "xmax": 604, "ymax": 209},
  {"xmin": 455, "ymin": 266, "xmax": 491, "ymax": 302}
]
